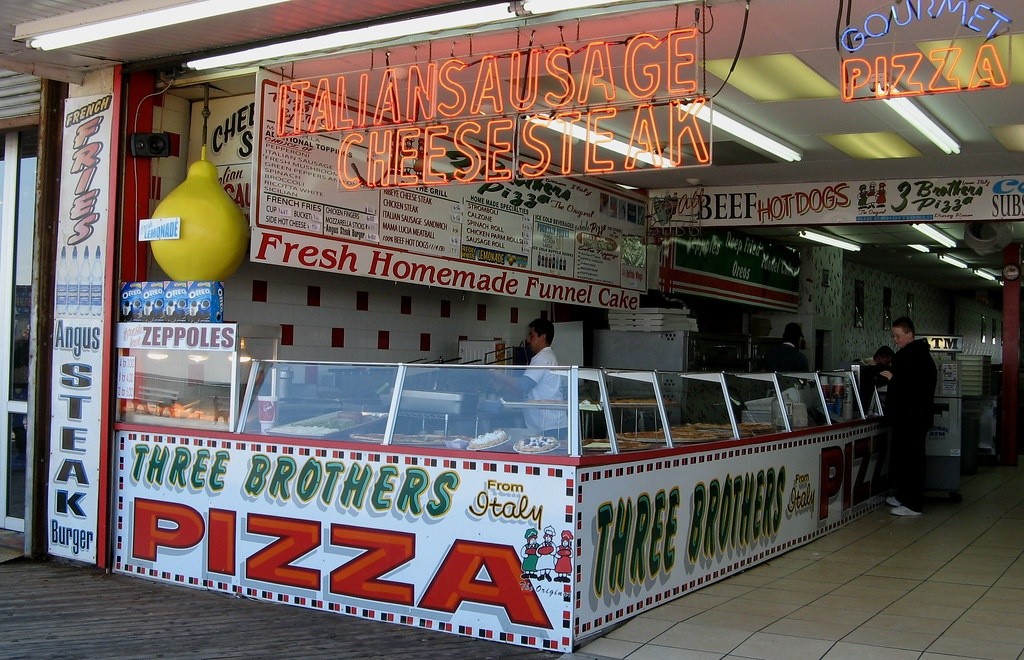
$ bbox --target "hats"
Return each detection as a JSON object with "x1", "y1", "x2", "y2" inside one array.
[{"x1": 784, "y1": 323, "x2": 804, "y2": 338}]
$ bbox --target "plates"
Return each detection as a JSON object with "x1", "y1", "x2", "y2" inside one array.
[
  {"x1": 513, "y1": 441, "x2": 561, "y2": 454},
  {"x1": 466, "y1": 434, "x2": 512, "y2": 450}
]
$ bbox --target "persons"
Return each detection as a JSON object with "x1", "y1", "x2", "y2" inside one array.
[
  {"x1": 880, "y1": 316, "x2": 938, "y2": 515},
  {"x1": 766, "y1": 322, "x2": 810, "y2": 373},
  {"x1": 860, "y1": 346, "x2": 895, "y2": 366},
  {"x1": 489, "y1": 318, "x2": 567, "y2": 439}
]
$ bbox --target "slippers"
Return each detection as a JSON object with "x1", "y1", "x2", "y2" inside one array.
[
  {"x1": 890, "y1": 505, "x2": 922, "y2": 516},
  {"x1": 885, "y1": 496, "x2": 901, "y2": 506}
]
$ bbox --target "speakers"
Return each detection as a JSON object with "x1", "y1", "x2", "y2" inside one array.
[{"x1": 130, "y1": 133, "x2": 169, "y2": 158}]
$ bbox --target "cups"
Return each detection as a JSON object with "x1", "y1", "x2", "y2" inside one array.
[{"x1": 258, "y1": 396, "x2": 279, "y2": 433}]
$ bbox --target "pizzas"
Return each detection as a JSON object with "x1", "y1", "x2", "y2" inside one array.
[
  {"x1": 524, "y1": 399, "x2": 567, "y2": 404},
  {"x1": 582, "y1": 422, "x2": 773, "y2": 449},
  {"x1": 350, "y1": 432, "x2": 469, "y2": 444},
  {"x1": 588, "y1": 399, "x2": 672, "y2": 405}
]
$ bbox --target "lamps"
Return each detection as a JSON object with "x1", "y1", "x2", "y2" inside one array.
[
  {"x1": 972, "y1": 268, "x2": 995, "y2": 281},
  {"x1": 180, "y1": 0, "x2": 619, "y2": 72},
  {"x1": 936, "y1": 253, "x2": 971, "y2": 269},
  {"x1": 187, "y1": 351, "x2": 209, "y2": 363},
  {"x1": 147, "y1": 350, "x2": 170, "y2": 360},
  {"x1": 228, "y1": 338, "x2": 252, "y2": 362},
  {"x1": 795, "y1": 227, "x2": 861, "y2": 252},
  {"x1": 867, "y1": 73, "x2": 962, "y2": 155},
  {"x1": 909, "y1": 223, "x2": 957, "y2": 248},
  {"x1": 673, "y1": 92, "x2": 806, "y2": 164},
  {"x1": 520, "y1": 111, "x2": 678, "y2": 168},
  {"x1": 907, "y1": 245, "x2": 930, "y2": 253},
  {"x1": 12, "y1": 0, "x2": 292, "y2": 52}
]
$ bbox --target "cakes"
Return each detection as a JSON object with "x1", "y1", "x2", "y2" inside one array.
[
  {"x1": 468, "y1": 430, "x2": 507, "y2": 449},
  {"x1": 514, "y1": 436, "x2": 557, "y2": 452}
]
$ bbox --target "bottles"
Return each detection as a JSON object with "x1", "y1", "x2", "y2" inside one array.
[
  {"x1": 653, "y1": 191, "x2": 678, "y2": 226},
  {"x1": 770, "y1": 392, "x2": 793, "y2": 427}
]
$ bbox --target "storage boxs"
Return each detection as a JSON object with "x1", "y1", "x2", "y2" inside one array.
[
  {"x1": 119, "y1": 280, "x2": 225, "y2": 323},
  {"x1": 608, "y1": 308, "x2": 698, "y2": 333},
  {"x1": 955, "y1": 355, "x2": 992, "y2": 395}
]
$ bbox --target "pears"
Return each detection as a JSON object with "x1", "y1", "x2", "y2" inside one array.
[{"x1": 149, "y1": 145, "x2": 248, "y2": 282}]
